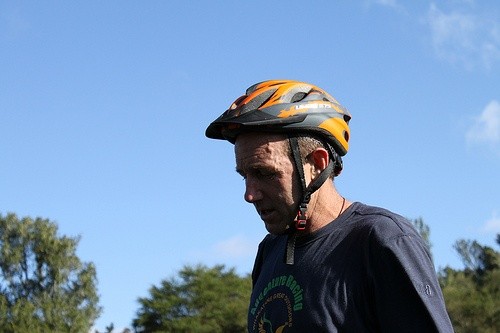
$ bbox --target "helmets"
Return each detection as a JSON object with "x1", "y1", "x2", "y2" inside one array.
[{"x1": 205, "y1": 80, "x2": 352, "y2": 155}]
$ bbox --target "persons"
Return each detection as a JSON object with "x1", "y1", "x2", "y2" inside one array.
[{"x1": 204, "y1": 81, "x2": 455, "y2": 330}]
